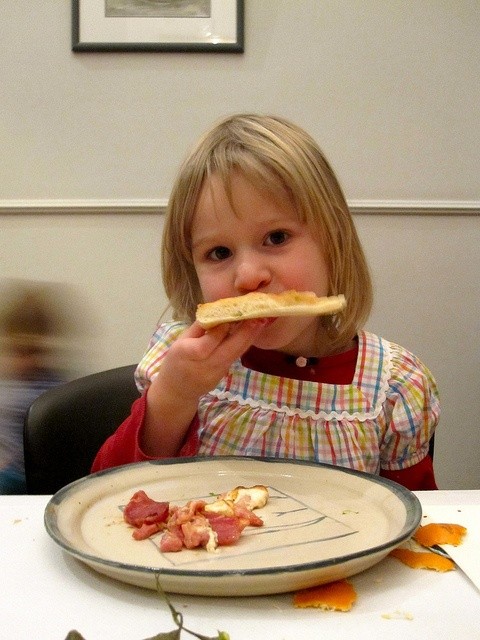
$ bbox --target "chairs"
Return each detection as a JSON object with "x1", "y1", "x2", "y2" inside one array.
[{"x1": 24, "y1": 364, "x2": 434, "y2": 494}]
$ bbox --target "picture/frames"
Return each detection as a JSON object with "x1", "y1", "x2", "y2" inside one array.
[{"x1": 71, "y1": 0, "x2": 245, "y2": 54}]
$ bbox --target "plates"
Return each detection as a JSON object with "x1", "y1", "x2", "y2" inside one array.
[{"x1": 43, "y1": 455, "x2": 423, "y2": 598}]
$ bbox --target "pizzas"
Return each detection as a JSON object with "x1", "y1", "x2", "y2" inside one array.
[{"x1": 196, "y1": 289, "x2": 348, "y2": 328}]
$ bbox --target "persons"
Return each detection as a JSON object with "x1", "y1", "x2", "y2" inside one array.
[{"x1": 89, "y1": 112, "x2": 439, "y2": 490}]
